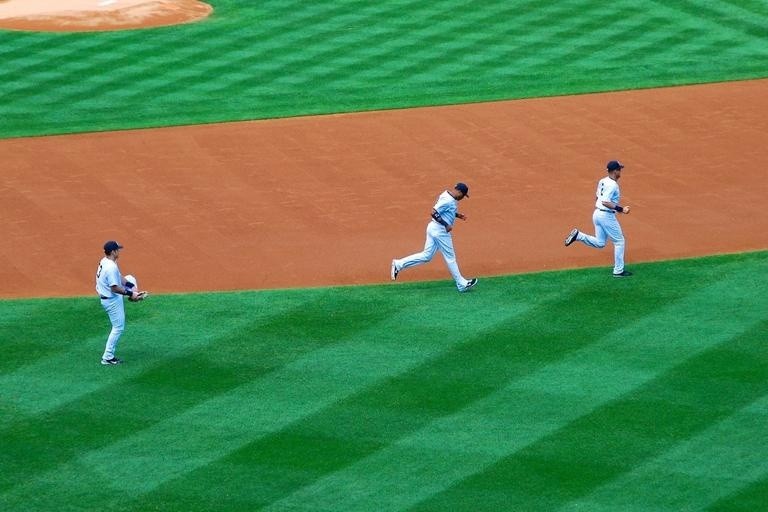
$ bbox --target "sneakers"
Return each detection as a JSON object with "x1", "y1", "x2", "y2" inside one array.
[
  {"x1": 460, "y1": 278, "x2": 479, "y2": 294},
  {"x1": 100, "y1": 358, "x2": 120, "y2": 365},
  {"x1": 129, "y1": 291, "x2": 146, "y2": 302},
  {"x1": 391, "y1": 260, "x2": 400, "y2": 282},
  {"x1": 565, "y1": 228, "x2": 578, "y2": 247},
  {"x1": 614, "y1": 270, "x2": 631, "y2": 277}
]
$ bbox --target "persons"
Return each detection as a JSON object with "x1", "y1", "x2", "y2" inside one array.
[
  {"x1": 96, "y1": 240, "x2": 147, "y2": 365},
  {"x1": 564, "y1": 160, "x2": 634, "y2": 277},
  {"x1": 391, "y1": 182, "x2": 478, "y2": 291}
]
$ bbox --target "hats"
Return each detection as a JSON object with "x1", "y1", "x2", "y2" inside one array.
[
  {"x1": 104, "y1": 241, "x2": 123, "y2": 251},
  {"x1": 454, "y1": 183, "x2": 469, "y2": 198},
  {"x1": 608, "y1": 161, "x2": 624, "y2": 172}
]
